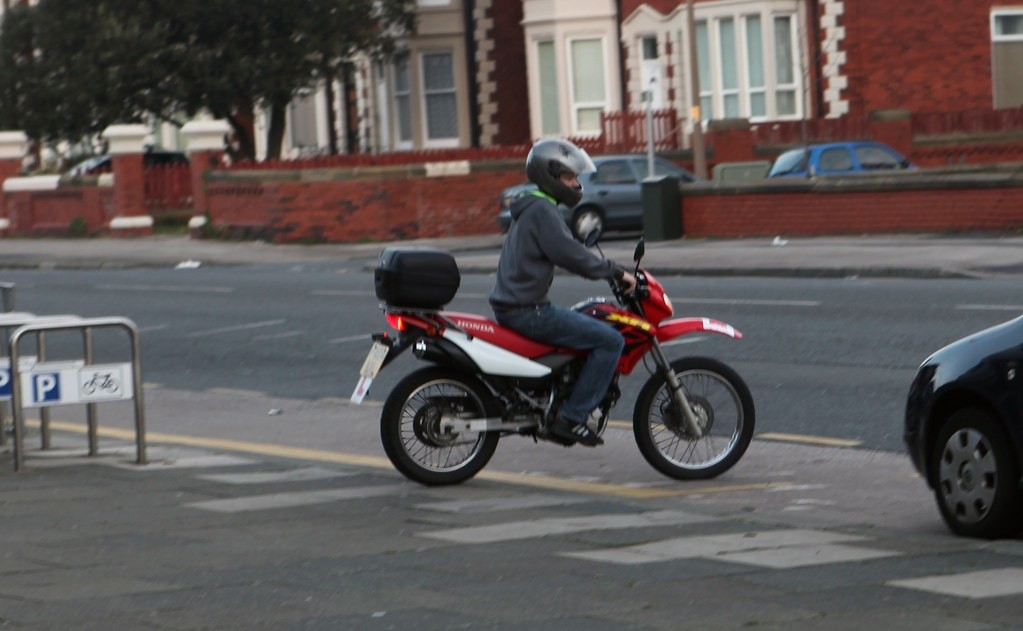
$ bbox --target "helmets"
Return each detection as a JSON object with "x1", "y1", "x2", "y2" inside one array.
[{"x1": 525, "y1": 140, "x2": 585, "y2": 208}]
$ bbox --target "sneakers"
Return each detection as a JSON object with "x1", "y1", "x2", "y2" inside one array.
[{"x1": 548, "y1": 414, "x2": 604, "y2": 446}]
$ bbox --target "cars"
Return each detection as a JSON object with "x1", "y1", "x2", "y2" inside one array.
[
  {"x1": 765, "y1": 139, "x2": 913, "y2": 181},
  {"x1": 901, "y1": 312, "x2": 1022, "y2": 539},
  {"x1": 498, "y1": 152, "x2": 696, "y2": 248},
  {"x1": 62, "y1": 152, "x2": 193, "y2": 227}
]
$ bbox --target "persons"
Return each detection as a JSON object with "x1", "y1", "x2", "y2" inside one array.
[{"x1": 489, "y1": 136, "x2": 637, "y2": 445}]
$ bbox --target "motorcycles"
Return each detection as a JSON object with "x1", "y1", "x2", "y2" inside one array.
[{"x1": 351, "y1": 228, "x2": 754, "y2": 488}]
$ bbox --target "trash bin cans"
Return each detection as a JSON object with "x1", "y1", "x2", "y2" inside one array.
[{"x1": 639, "y1": 173, "x2": 683, "y2": 242}]
